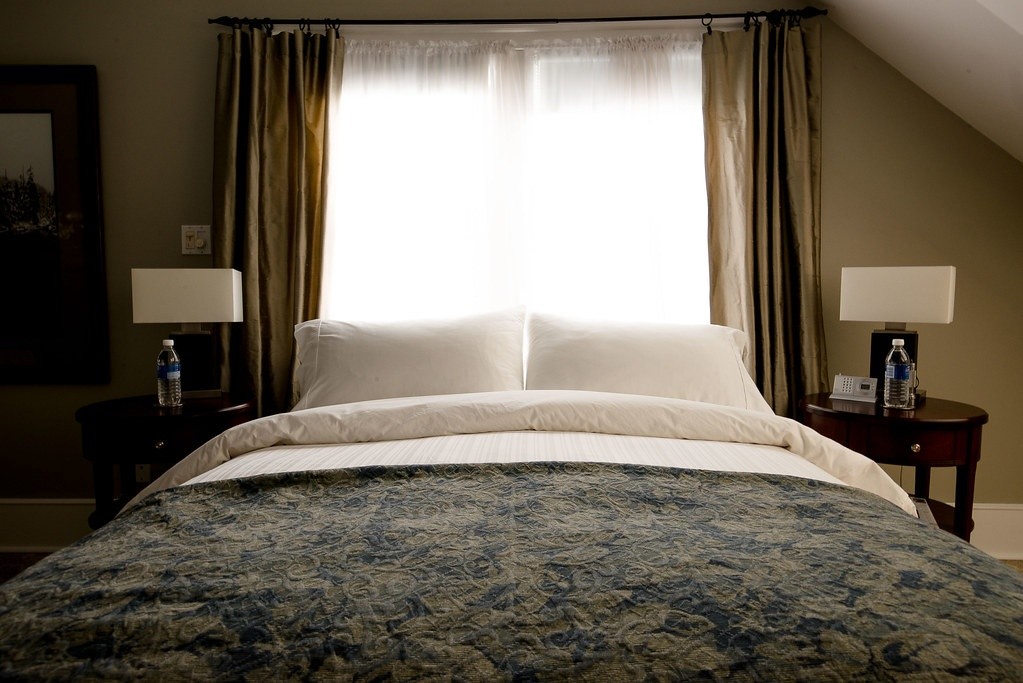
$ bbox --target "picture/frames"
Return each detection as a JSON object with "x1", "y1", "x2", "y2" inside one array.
[{"x1": 0, "y1": 64, "x2": 113, "y2": 389}]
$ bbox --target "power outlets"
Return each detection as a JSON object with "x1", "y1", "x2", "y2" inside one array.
[{"x1": 181, "y1": 225, "x2": 213, "y2": 256}]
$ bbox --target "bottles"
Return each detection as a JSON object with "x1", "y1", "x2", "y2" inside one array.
[
  {"x1": 157, "y1": 339, "x2": 182, "y2": 408},
  {"x1": 883, "y1": 338, "x2": 910, "y2": 407},
  {"x1": 909, "y1": 362, "x2": 915, "y2": 408}
]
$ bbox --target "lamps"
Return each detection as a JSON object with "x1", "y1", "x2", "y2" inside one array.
[
  {"x1": 132, "y1": 268, "x2": 244, "y2": 398},
  {"x1": 839, "y1": 266, "x2": 956, "y2": 402}
]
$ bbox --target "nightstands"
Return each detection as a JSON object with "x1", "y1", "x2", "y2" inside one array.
[
  {"x1": 798, "y1": 393, "x2": 990, "y2": 544},
  {"x1": 76, "y1": 395, "x2": 250, "y2": 534}
]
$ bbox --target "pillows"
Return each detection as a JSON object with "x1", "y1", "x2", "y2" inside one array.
[
  {"x1": 288, "y1": 303, "x2": 526, "y2": 413},
  {"x1": 526, "y1": 310, "x2": 776, "y2": 417}
]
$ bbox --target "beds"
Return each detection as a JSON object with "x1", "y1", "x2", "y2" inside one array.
[{"x1": 0, "y1": 389, "x2": 1023, "y2": 683}]
